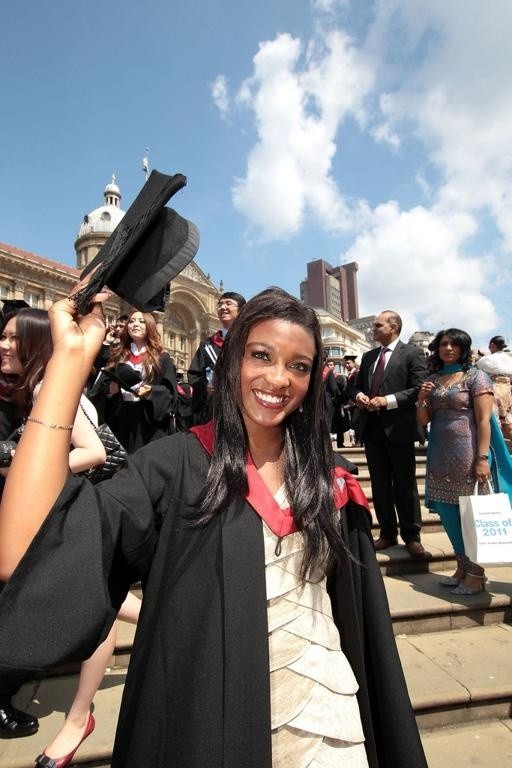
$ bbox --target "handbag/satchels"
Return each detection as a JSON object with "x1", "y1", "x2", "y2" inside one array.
[
  {"x1": 66, "y1": 402, "x2": 133, "y2": 493},
  {"x1": 458, "y1": 472, "x2": 512, "y2": 569}
]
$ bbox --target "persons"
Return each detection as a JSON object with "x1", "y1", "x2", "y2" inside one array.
[
  {"x1": 187, "y1": 292, "x2": 245, "y2": 421},
  {"x1": 109, "y1": 311, "x2": 177, "y2": 452},
  {"x1": 0, "y1": 297, "x2": 141, "y2": 766},
  {"x1": 172, "y1": 373, "x2": 193, "y2": 431},
  {"x1": 323, "y1": 309, "x2": 512, "y2": 596},
  {"x1": 0, "y1": 259, "x2": 428, "y2": 767}
]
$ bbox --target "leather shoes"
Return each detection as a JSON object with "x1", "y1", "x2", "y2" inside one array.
[
  {"x1": 438, "y1": 575, "x2": 460, "y2": 588},
  {"x1": 373, "y1": 535, "x2": 399, "y2": 551},
  {"x1": 404, "y1": 541, "x2": 425, "y2": 556},
  {"x1": 0, "y1": 694, "x2": 40, "y2": 739},
  {"x1": 35, "y1": 708, "x2": 96, "y2": 768},
  {"x1": 449, "y1": 579, "x2": 485, "y2": 595}
]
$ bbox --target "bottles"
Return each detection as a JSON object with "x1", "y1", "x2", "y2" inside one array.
[{"x1": 205, "y1": 366, "x2": 214, "y2": 384}]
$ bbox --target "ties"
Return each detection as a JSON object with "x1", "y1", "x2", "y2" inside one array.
[{"x1": 364, "y1": 349, "x2": 392, "y2": 401}]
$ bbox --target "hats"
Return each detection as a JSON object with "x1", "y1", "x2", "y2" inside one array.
[
  {"x1": 97, "y1": 362, "x2": 146, "y2": 401},
  {"x1": 67, "y1": 166, "x2": 208, "y2": 325},
  {"x1": 341, "y1": 353, "x2": 358, "y2": 362}
]
